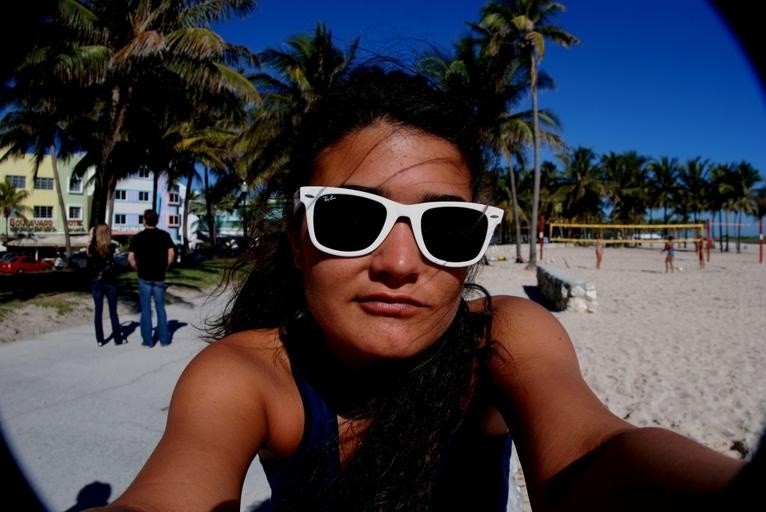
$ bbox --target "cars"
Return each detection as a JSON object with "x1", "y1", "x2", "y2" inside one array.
[{"x1": 0, "y1": 255, "x2": 55, "y2": 274}]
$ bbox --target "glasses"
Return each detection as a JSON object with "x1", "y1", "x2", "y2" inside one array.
[{"x1": 295, "y1": 185, "x2": 505, "y2": 270}]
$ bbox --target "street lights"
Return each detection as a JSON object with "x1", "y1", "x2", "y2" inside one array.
[{"x1": 239, "y1": 180, "x2": 250, "y2": 254}]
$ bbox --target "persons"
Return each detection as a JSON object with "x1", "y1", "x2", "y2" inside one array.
[
  {"x1": 84, "y1": 222, "x2": 130, "y2": 345},
  {"x1": 595, "y1": 231, "x2": 603, "y2": 269},
  {"x1": 662, "y1": 235, "x2": 675, "y2": 274},
  {"x1": 63, "y1": 51, "x2": 754, "y2": 512},
  {"x1": 124, "y1": 208, "x2": 178, "y2": 347}
]
